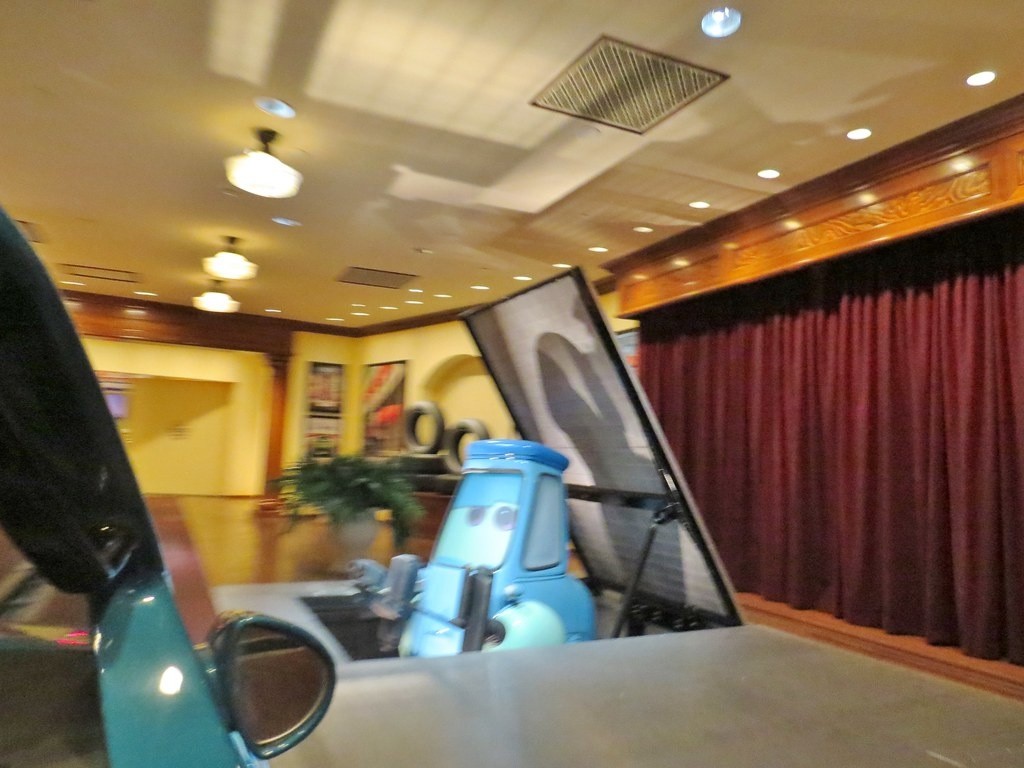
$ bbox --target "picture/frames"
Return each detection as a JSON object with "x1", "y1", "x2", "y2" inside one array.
[
  {"x1": 310, "y1": 362, "x2": 345, "y2": 412},
  {"x1": 302, "y1": 416, "x2": 341, "y2": 458},
  {"x1": 362, "y1": 359, "x2": 406, "y2": 452}
]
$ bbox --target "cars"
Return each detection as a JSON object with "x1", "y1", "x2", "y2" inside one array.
[{"x1": 0, "y1": 211, "x2": 339, "y2": 767}]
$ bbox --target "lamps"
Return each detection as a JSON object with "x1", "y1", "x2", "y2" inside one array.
[
  {"x1": 191, "y1": 282, "x2": 239, "y2": 313},
  {"x1": 202, "y1": 237, "x2": 259, "y2": 281},
  {"x1": 224, "y1": 127, "x2": 302, "y2": 199}
]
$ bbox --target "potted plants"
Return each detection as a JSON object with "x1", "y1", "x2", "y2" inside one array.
[{"x1": 262, "y1": 450, "x2": 428, "y2": 548}]
